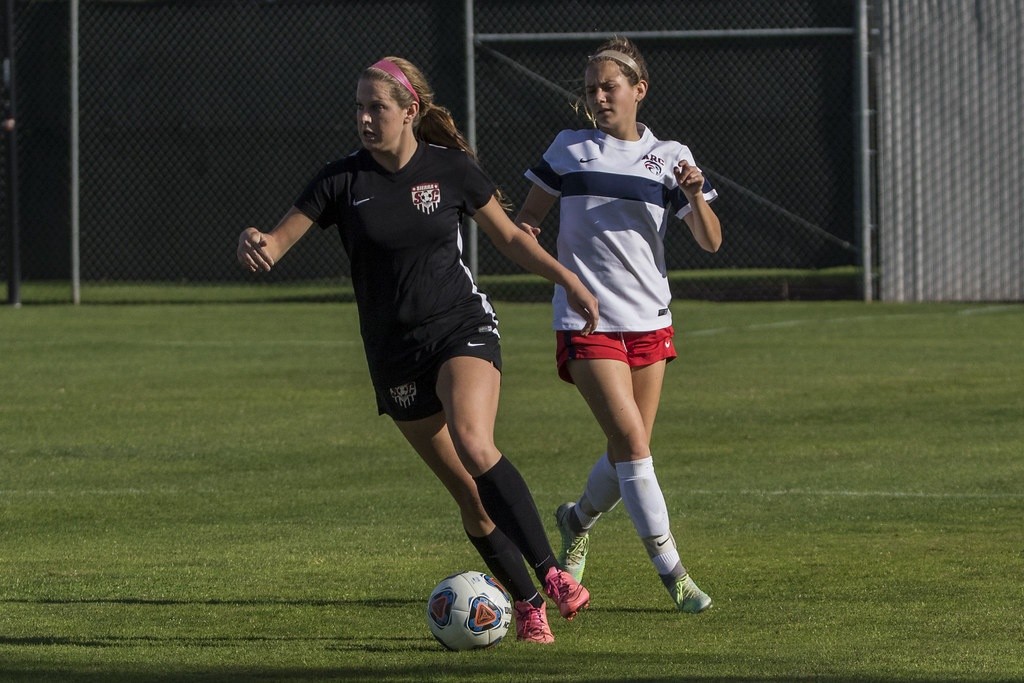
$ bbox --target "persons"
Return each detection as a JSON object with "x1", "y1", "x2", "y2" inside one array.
[
  {"x1": 233, "y1": 52, "x2": 601, "y2": 649},
  {"x1": 505, "y1": 29, "x2": 728, "y2": 616}
]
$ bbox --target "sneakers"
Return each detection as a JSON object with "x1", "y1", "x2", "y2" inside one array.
[
  {"x1": 543, "y1": 567, "x2": 590, "y2": 621},
  {"x1": 666, "y1": 571, "x2": 712, "y2": 614},
  {"x1": 554, "y1": 501, "x2": 589, "y2": 584},
  {"x1": 512, "y1": 600, "x2": 555, "y2": 643}
]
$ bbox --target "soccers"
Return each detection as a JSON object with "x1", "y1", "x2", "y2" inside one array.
[{"x1": 427, "y1": 571, "x2": 511, "y2": 652}]
